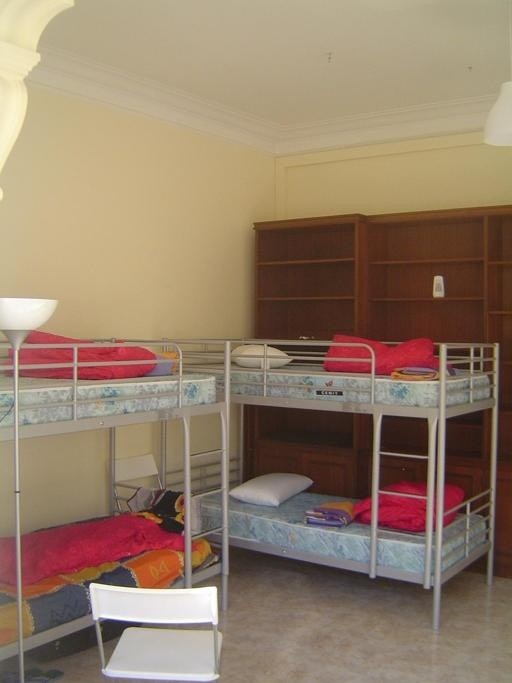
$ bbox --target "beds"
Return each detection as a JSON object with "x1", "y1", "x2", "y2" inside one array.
[
  {"x1": 164, "y1": 335, "x2": 500, "y2": 631},
  {"x1": 0, "y1": 338, "x2": 233, "y2": 682}
]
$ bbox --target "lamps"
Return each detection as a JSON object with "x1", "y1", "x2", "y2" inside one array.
[
  {"x1": 0, "y1": 296, "x2": 61, "y2": 683},
  {"x1": 482, "y1": 79, "x2": 512, "y2": 148}
]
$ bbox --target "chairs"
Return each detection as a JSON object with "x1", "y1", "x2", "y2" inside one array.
[
  {"x1": 102, "y1": 451, "x2": 166, "y2": 514},
  {"x1": 87, "y1": 582, "x2": 223, "y2": 682}
]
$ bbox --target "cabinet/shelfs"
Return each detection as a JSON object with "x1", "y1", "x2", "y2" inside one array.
[{"x1": 244, "y1": 202, "x2": 511, "y2": 578}]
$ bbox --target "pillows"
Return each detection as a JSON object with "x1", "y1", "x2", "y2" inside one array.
[
  {"x1": 229, "y1": 470, "x2": 314, "y2": 508},
  {"x1": 232, "y1": 342, "x2": 289, "y2": 366}
]
